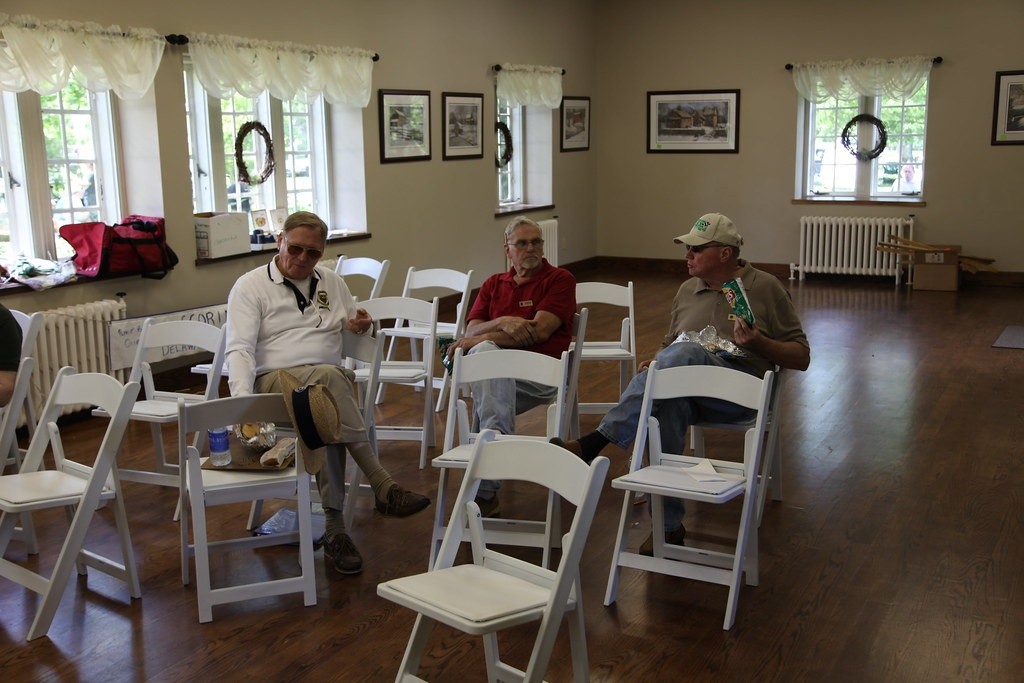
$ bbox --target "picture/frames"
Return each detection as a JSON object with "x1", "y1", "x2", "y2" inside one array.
[
  {"x1": 559, "y1": 95, "x2": 590, "y2": 153},
  {"x1": 441, "y1": 91, "x2": 484, "y2": 161},
  {"x1": 991, "y1": 71, "x2": 1024, "y2": 147},
  {"x1": 645, "y1": 89, "x2": 741, "y2": 154},
  {"x1": 377, "y1": 88, "x2": 432, "y2": 165}
]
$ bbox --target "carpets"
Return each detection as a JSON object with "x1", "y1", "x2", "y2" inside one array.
[{"x1": 991, "y1": 325, "x2": 1024, "y2": 348}]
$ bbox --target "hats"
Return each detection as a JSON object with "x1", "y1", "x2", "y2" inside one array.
[
  {"x1": 673, "y1": 213, "x2": 744, "y2": 247},
  {"x1": 277, "y1": 368, "x2": 341, "y2": 474}
]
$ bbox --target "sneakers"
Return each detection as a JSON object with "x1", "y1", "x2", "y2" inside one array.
[
  {"x1": 323, "y1": 532, "x2": 363, "y2": 575},
  {"x1": 374, "y1": 483, "x2": 433, "y2": 520}
]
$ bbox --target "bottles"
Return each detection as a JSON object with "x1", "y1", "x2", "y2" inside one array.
[{"x1": 208, "y1": 425, "x2": 231, "y2": 466}]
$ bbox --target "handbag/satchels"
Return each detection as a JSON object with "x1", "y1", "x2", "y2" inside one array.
[{"x1": 59, "y1": 214, "x2": 180, "y2": 280}]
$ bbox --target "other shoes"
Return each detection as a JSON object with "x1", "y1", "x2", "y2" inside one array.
[
  {"x1": 639, "y1": 523, "x2": 686, "y2": 556},
  {"x1": 474, "y1": 491, "x2": 501, "y2": 518},
  {"x1": 549, "y1": 437, "x2": 593, "y2": 466}
]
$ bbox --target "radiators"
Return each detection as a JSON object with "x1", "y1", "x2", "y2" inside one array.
[
  {"x1": 790, "y1": 215, "x2": 914, "y2": 285},
  {"x1": 506, "y1": 219, "x2": 558, "y2": 274},
  {"x1": 17, "y1": 292, "x2": 130, "y2": 429}
]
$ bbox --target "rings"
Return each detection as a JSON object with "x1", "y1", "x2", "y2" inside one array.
[{"x1": 357, "y1": 329, "x2": 363, "y2": 334}]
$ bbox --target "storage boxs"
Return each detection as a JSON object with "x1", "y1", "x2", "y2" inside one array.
[
  {"x1": 193, "y1": 211, "x2": 252, "y2": 259},
  {"x1": 914, "y1": 244, "x2": 961, "y2": 291}
]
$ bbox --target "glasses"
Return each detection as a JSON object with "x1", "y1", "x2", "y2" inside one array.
[
  {"x1": 284, "y1": 233, "x2": 324, "y2": 260},
  {"x1": 507, "y1": 238, "x2": 544, "y2": 250},
  {"x1": 302, "y1": 294, "x2": 323, "y2": 328},
  {"x1": 686, "y1": 243, "x2": 732, "y2": 253}
]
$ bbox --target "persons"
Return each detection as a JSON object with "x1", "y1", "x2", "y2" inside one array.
[
  {"x1": 814, "y1": 149, "x2": 825, "y2": 185},
  {"x1": 0, "y1": 301, "x2": 23, "y2": 408},
  {"x1": 71, "y1": 163, "x2": 99, "y2": 222},
  {"x1": 890, "y1": 165, "x2": 919, "y2": 192},
  {"x1": 317, "y1": 291, "x2": 329, "y2": 307},
  {"x1": 223, "y1": 211, "x2": 432, "y2": 575},
  {"x1": 446, "y1": 215, "x2": 576, "y2": 517},
  {"x1": 548, "y1": 212, "x2": 811, "y2": 555}
]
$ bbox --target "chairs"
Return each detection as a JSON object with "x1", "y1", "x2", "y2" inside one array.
[{"x1": 0, "y1": 257, "x2": 785, "y2": 683}]
